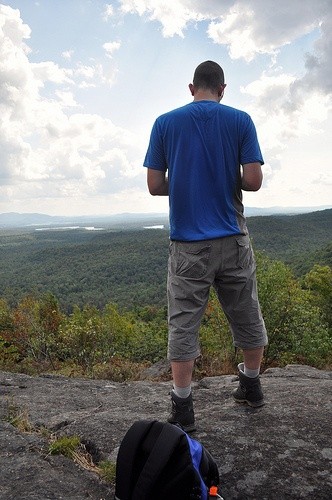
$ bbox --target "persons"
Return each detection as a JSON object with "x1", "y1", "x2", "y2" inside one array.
[{"x1": 143, "y1": 61, "x2": 269, "y2": 432}]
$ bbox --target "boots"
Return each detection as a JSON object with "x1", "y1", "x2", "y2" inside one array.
[
  {"x1": 164, "y1": 388, "x2": 196, "y2": 432},
  {"x1": 231, "y1": 362, "x2": 265, "y2": 408}
]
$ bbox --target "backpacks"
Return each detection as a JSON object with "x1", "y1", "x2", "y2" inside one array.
[{"x1": 114, "y1": 418, "x2": 223, "y2": 500}]
